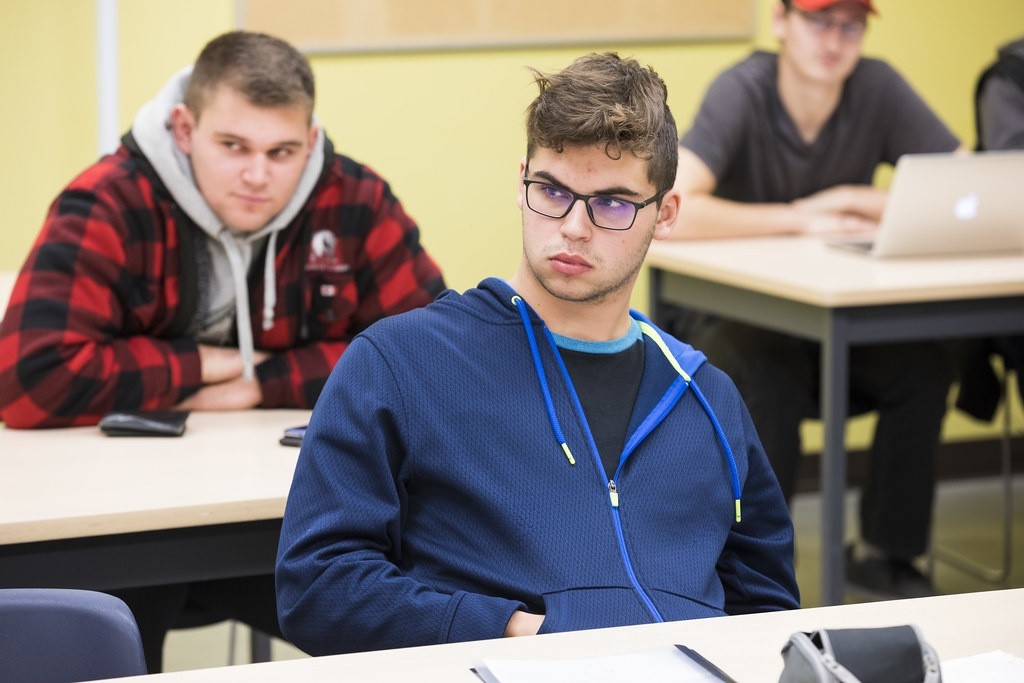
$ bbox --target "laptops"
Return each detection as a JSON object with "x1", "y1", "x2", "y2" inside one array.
[{"x1": 826, "y1": 149, "x2": 1024, "y2": 257}]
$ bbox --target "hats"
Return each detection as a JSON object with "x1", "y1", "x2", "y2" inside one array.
[{"x1": 789, "y1": 0, "x2": 882, "y2": 18}]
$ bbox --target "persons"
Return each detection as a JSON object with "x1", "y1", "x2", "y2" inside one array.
[
  {"x1": 660, "y1": 0, "x2": 972, "y2": 600},
  {"x1": 0, "y1": 32, "x2": 446, "y2": 673},
  {"x1": 275, "y1": 53, "x2": 802, "y2": 654}
]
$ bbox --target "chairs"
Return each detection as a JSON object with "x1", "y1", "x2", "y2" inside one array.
[{"x1": 0, "y1": 587, "x2": 148, "y2": 683}]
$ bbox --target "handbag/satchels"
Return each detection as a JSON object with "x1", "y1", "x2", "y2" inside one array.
[{"x1": 778, "y1": 626, "x2": 943, "y2": 683}]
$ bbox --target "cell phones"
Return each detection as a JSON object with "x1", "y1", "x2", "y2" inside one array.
[{"x1": 284, "y1": 425, "x2": 308, "y2": 438}]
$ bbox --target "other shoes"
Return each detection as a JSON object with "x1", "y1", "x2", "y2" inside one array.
[{"x1": 844, "y1": 540, "x2": 935, "y2": 598}]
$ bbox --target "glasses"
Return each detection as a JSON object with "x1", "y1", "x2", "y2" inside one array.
[
  {"x1": 789, "y1": 6, "x2": 867, "y2": 37},
  {"x1": 523, "y1": 162, "x2": 671, "y2": 230}
]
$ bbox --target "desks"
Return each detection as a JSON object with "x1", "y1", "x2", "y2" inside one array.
[
  {"x1": 0, "y1": 409, "x2": 313, "y2": 663},
  {"x1": 649, "y1": 235, "x2": 1024, "y2": 606},
  {"x1": 108, "y1": 586, "x2": 1023, "y2": 683}
]
